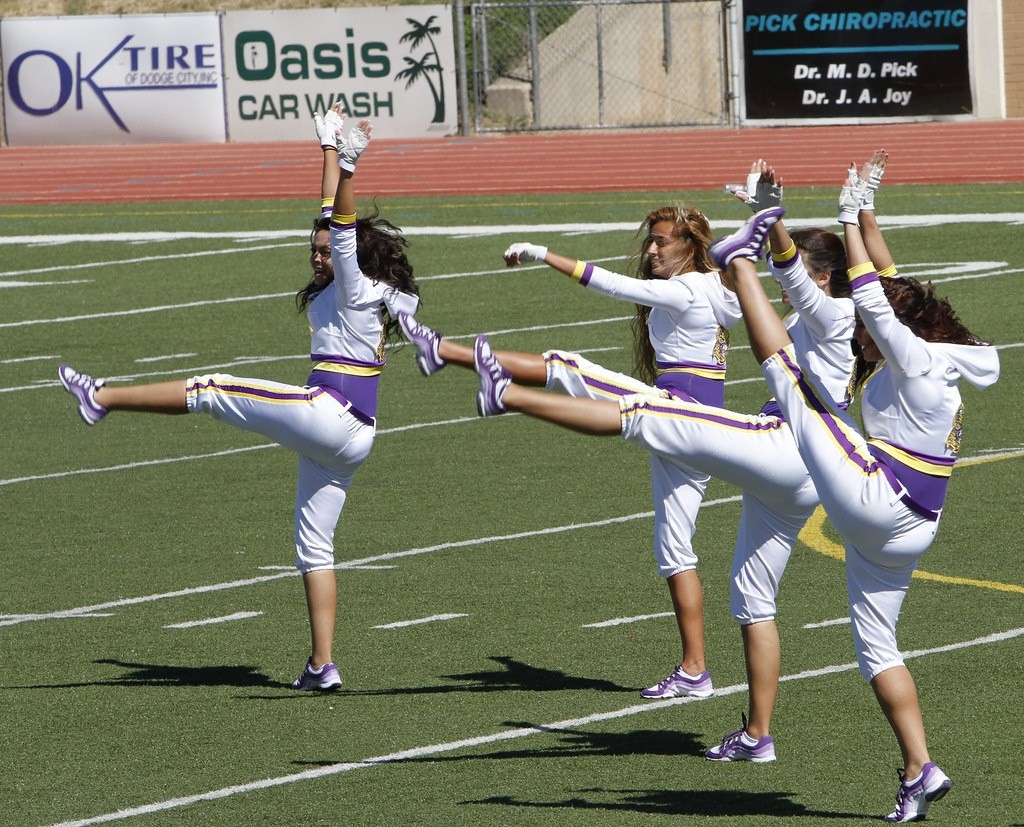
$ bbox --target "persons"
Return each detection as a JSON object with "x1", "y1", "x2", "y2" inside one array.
[
  {"x1": 396, "y1": 147, "x2": 1001, "y2": 824},
  {"x1": 57, "y1": 101, "x2": 425, "y2": 692}
]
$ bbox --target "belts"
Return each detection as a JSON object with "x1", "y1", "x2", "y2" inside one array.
[
  {"x1": 879, "y1": 464, "x2": 938, "y2": 521},
  {"x1": 667, "y1": 385, "x2": 696, "y2": 405},
  {"x1": 322, "y1": 387, "x2": 375, "y2": 427}
]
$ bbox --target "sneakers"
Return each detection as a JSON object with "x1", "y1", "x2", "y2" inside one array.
[
  {"x1": 473, "y1": 334, "x2": 513, "y2": 417},
  {"x1": 706, "y1": 205, "x2": 786, "y2": 272},
  {"x1": 884, "y1": 762, "x2": 951, "y2": 824},
  {"x1": 396, "y1": 310, "x2": 447, "y2": 377},
  {"x1": 704, "y1": 712, "x2": 776, "y2": 764},
  {"x1": 58, "y1": 364, "x2": 110, "y2": 426},
  {"x1": 640, "y1": 664, "x2": 715, "y2": 699},
  {"x1": 291, "y1": 656, "x2": 342, "y2": 691}
]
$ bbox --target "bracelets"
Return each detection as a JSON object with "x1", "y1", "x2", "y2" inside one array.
[{"x1": 323, "y1": 147, "x2": 337, "y2": 152}]
